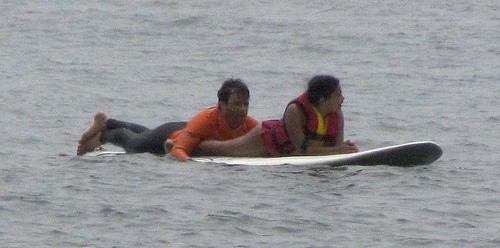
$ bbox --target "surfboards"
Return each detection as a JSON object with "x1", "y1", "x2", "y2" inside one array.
[{"x1": 81, "y1": 141, "x2": 442, "y2": 169}]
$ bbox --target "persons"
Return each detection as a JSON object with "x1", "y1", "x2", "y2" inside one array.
[
  {"x1": 76, "y1": 75, "x2": 262, "y2": 165},
  {"x1": 164, "y1": 74, "x2": 361, "y2": 162}
]
